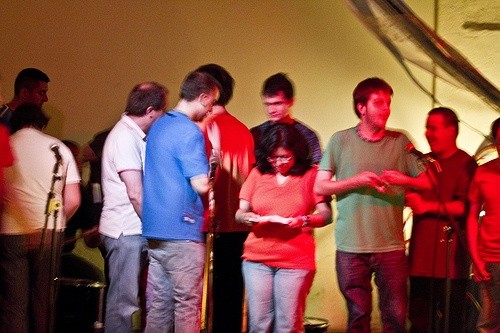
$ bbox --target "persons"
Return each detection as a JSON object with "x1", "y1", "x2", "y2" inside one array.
[
  {"x1": 405, "y1": 108, "x2": 478, "y2": 333},
  {"x1": 313, "y1": 77, "x2": 433, "y2": 333},
  {"x1": 0, "y1": 68, "x2": 116, "y2": 333},
  {"x1": 142, "y1": 70, "x2": 220, "y2": 333},
  {"x1": 196, "y1": 63, "x2": 323, "y2": 333},
  {"x1": 99, "y1": 81, "x2": 169, "y2": 333},
  {"x1": 236, "y1": 123, "x2": 333, "y2": 333},
  {"x1": 466, "y1": 118, "x2": 500, "y2": 333}
]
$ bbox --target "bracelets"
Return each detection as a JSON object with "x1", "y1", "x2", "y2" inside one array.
[{"x1": 301, "y1": 215, "x2": 310, "y2": 228}]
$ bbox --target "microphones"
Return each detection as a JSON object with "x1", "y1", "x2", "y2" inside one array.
[
  {"x1": 405, "y1": 144, "x2": 435, "y2": 162},
  {"x1": 51, "y1": 144, "x2": 62, "y2": 163},
  {"x1": 210, "y1": 156, "x2": 220, "y2": 180}
]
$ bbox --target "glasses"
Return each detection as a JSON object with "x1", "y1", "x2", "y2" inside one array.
[{"x1": 267, "y1": 155, "x2": 294, "y2": 163}]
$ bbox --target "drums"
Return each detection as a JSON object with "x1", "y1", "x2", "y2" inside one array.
[{"x1": 272, "y1": 317, "x2": 329, "y2": 333}]
